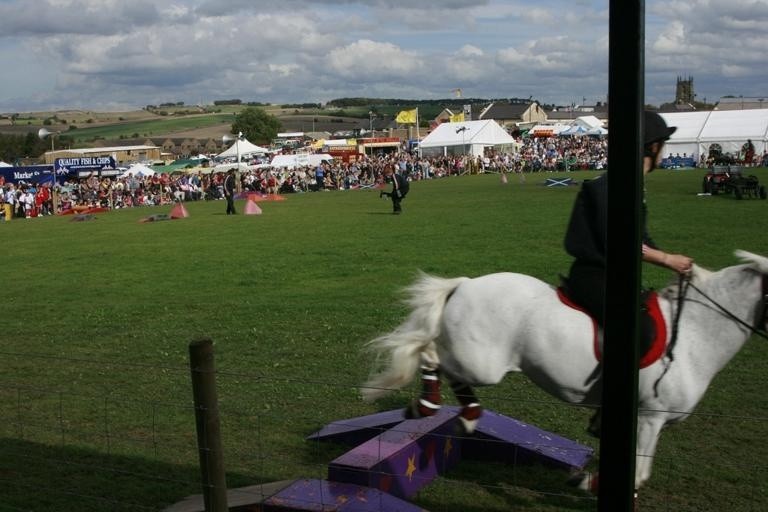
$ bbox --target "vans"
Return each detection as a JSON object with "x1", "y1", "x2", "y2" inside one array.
[{"x1": 271, "y1": 153, "x2": 336, "y2": 168}]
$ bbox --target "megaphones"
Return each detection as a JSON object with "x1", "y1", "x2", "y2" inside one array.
[
  {"x1": 359, "y1": 128, "x2": 372, "y2": 136},
  {"x1": 455, "y1": 128, "x2": 463, "y2": 134},
  {"x1": 38, "y1": 127, "x2": 61, "y2": 141},
  {"x1": 222, "y1": 135, "x2": 237, "y2": 144}
]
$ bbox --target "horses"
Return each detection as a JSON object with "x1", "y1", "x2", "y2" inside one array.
[{"x1": 358, "y1": 249, "x2": 768, "y2": 498}]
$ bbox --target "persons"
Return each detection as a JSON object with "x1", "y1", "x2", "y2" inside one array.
[
  {"x1": 692, "y1": 154, "x2": 697, "y2": 168},
  {"x1": 675, "y1": 153, "x2": 681, "y2": 158},
  {"x1": 668, "y1": 153, "x2": 674, "y2": 159},
  {"x1": 702, "y1": 139, "x2": 768, "y2": 167},
  {"x1": 561, "y1": 113, "x2": 692, "y2": 439},
  {"x1": 682, "y1": 153, "x2": 688, "y2": 158}
]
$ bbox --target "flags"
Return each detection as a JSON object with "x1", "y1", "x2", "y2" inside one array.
[
  {"x1": 450, "y1": 112, "x2": 464, "y2": 123},
  {"x1": 395, "y1": 108, "x2": 417, "y2": 124}
]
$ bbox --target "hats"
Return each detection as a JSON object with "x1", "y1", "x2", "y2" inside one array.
[
  {"x1": 228, "y1": 167, "x2": 238, "y2": 174},
  {"x1": 640, "y1": 109, "x2": 677, "y2": 144}
]
